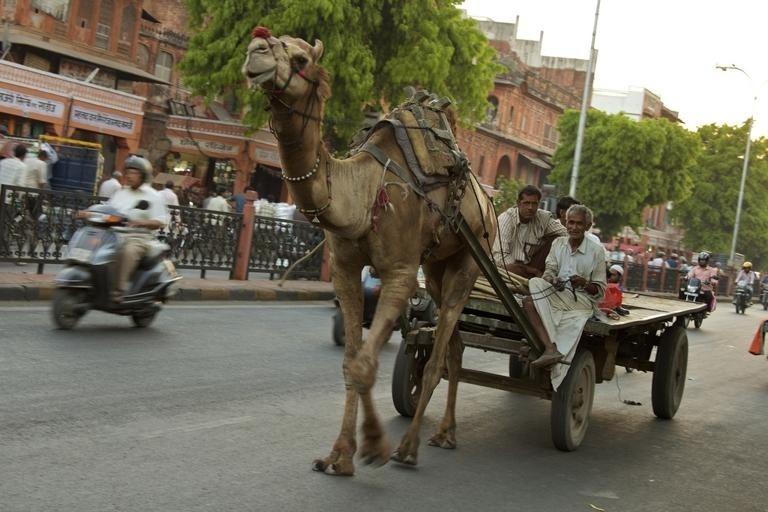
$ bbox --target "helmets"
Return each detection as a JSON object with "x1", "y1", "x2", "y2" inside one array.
[
  {"x1": 123, "y1": 155, "x2": 153, "y2": 182},
  {"x1": 742, "y1": 261, "x2": 752, "y2": 270},
  {"x1": 697, "y1": 251, "x2": 709, "y2": 267}
]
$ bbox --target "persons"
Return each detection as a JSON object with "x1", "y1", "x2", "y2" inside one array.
[
  {"x1": 606, "y1": 246, "x2": 768, "y2": 313},
  {"x1": 79, "y1": 157, "x2": 180, "y2": 298},
  {"x1": 1, "y1": 145, "x2": 49, "y2": 256},
  {"x1": 205, "y1": 190, "x2": 308, "y2": 267},
  {"x1": 491, "y1": 186, "x2": 606, "y2": 390}
]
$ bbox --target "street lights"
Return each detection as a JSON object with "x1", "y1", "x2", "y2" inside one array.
[{"x1": 712, "y1": 60, "x2": 761, "y2": 272}]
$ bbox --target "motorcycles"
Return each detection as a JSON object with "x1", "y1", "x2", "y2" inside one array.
[
  {"x1": 49, "y1": 187, "x2": 182, "y2": 335},
  {"x1": 677, "y1": 273, "x2": 767, "y2": 333},
  {"x1": 330, "y1": 265, "x2": 400, "y2": 348}
]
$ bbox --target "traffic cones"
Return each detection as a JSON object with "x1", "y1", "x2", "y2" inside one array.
[{"x1": 746, "y1": 325, "x2": 761, "y2": 355}]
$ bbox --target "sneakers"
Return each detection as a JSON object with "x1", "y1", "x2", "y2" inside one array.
[{"x1": 530, "y1": 343, "x2": 564, "y2": 367}]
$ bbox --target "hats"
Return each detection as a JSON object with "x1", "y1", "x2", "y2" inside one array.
[{"x1": 610, "y1": 264, "x2": 624, "y2": 276}]
